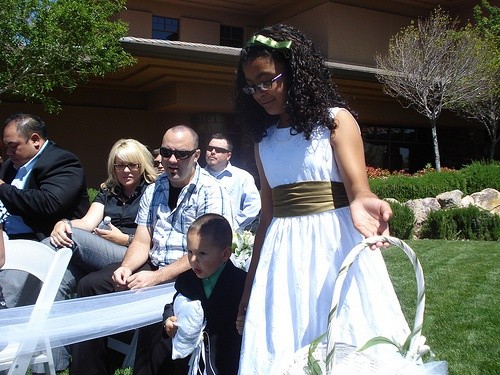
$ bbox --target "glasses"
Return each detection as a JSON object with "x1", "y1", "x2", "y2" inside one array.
[
  {"x1": 206, "y1": 146, "x2": 232, "y2": 153},
  {"x1": 114, "y1": 163, "x2": 139, "y2": 169},
  {"x1": 242, "y1": 73, "x2": 283, "y2": 96},
  {"x1": 160, "y1": 148, "x2": 196, "y2": 160},
  {"x1": 153, "y1": 160, "x2": 163, "y2": 167}
]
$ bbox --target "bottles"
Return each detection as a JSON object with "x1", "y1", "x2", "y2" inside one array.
[{"x1": 94, "y1": 216, "x2": 112, "y2": 234}]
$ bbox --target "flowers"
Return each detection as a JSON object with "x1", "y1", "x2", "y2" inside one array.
[
  {"x1": 232, "y1": 230, "x2": 256, "y2": 270},
  {"x1": 356, "y1": 325, "x2": 435, "y2": 366}
]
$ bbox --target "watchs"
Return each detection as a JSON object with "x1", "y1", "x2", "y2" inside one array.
[{"x1": 61, "y1": 218, "x2": 72, "y2": 229}]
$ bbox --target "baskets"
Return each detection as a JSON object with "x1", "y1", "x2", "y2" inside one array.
[{"x1": 283, "y1": 236, "x2": 448, "y2": 375}]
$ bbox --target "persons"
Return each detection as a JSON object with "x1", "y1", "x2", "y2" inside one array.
[
  {"x1": 0, "y1": 113, "x2": 91, "y2": 242},
  {"x1": 204, "y1": 132, "x2": 262, "y2": 232},
  {"x1": 162, "y1": 213, "x2": 248, "y2": 375},
  {"x1": 77, "y1": 125, "x2": 233, "y2": 298},
  {"x1": 151, "y1": 148, "x2": 164, "y2": 176},
  {"x1": 40, "y1": 138, "x2": 158, "y2": 301},
  {"x1": 236, "y1": 24, "x2": 394, "y2": 375}
]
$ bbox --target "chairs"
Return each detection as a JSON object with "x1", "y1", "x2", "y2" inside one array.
[{"x1": 0, "y1": 231, "x2": 73, "y2": 375}]
